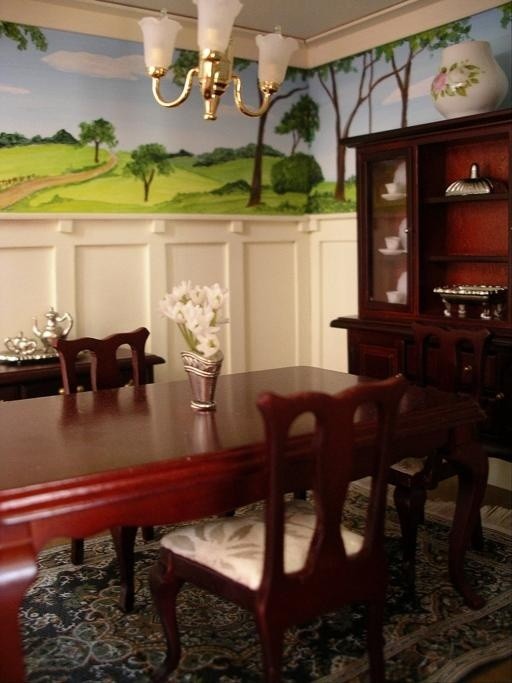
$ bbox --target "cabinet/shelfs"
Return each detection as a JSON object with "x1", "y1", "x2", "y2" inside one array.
[{"x1": 327, "y1": 106, "x2": 512, "y2": 465}]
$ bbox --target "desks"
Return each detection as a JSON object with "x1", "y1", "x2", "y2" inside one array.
[{"x1": 1, "y1": 342, "x2": 166, "y2": 402}]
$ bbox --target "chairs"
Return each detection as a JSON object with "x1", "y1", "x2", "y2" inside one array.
[
  {"x1": 365, "y1": 323, "x2": 493, "y2": 612},
  {"x1": 147, "y1": 374, "x2": 402, "y2": 680},
  {"x1": 50, "y1": 328, "x2": 152, "y2": 614}
]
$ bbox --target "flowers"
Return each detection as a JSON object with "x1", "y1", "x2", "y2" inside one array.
[{"x1": 158, "y1": 281, "x2": 237, "y2": 363}]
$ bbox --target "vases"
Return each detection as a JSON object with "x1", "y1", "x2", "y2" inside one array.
[
  {"x1": 175, "y1": 350, "x2": 225, "y2": 414},
  {"x1": 431, "y1": 36, "x2": 508, "y2": 119}
]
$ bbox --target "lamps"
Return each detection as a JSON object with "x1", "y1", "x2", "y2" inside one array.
[{"x1": 136, "y1": 0, "x2": 301, "y2": 123}]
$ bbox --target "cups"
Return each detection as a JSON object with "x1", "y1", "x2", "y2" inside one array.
[
  {"x1": 383, "y1": 235, "x2": 401, "y2": 248},
  {"x1": 385, "y1": 290, "x2": 406, "y2": 304},
  {"x1": 384, "y1": 182, "x2": 402, "y2": 192}
]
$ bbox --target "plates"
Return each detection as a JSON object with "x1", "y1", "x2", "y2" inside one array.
[
  {"x1": 397, "y1": 270, "x2": 407, "y2": 294},
  {"x1": 398, "y1": 217, "x2": 407, "y2": 249},
  {"x1": 376, "y1": 247, "x2": 404, "y2": 256},
  {"x1": 392, "y1": 161, "x2": 406, "y2": 190},
  {"x1": 381, "y1": 192, "x2": 406, "y2": 200}
]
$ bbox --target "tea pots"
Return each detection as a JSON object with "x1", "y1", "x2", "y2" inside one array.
[
  {"x1": 31, "y1": 304, "x2": 73, "y2": 354},
  {"x1": 4, "y1": 330, "x2": 38, "y2": 356}
]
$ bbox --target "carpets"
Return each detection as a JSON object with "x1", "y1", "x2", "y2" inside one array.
[{"x1": 18, "y1": 480, "x2": 510, "y2": 682}]
